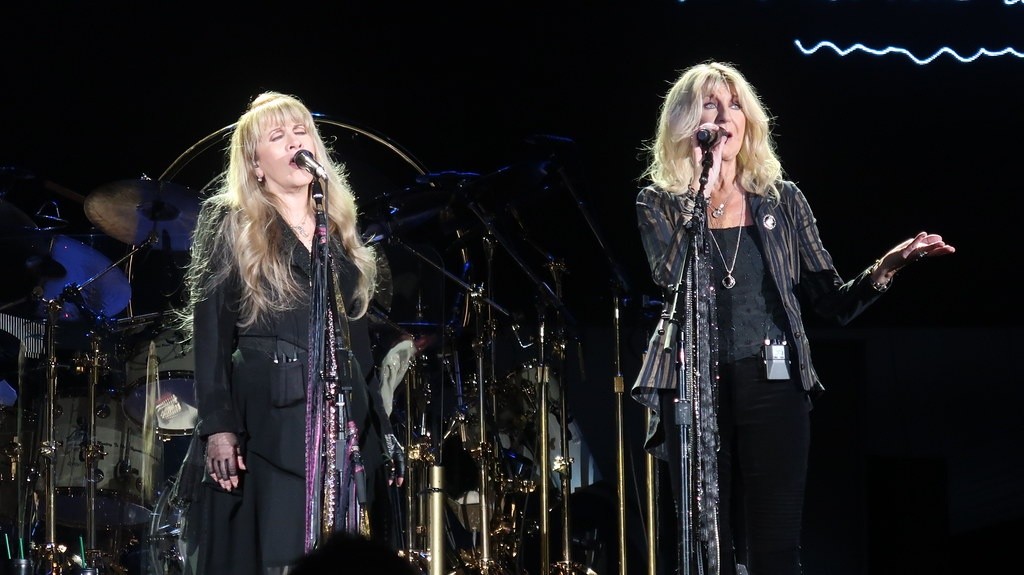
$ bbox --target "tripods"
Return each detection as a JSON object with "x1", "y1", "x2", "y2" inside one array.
[
  {"x1": 0, "y1": 223, "x2": 161, "y2": 575},
  {"x1": 377, "y1": 188, "x2": 598, "y2": 575}
]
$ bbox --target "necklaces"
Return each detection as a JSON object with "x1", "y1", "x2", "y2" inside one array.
[
  {"x1": 287, "y1": 212, "x2": 312, "y2": 239},
  {"x1": 706, "y1": 183, "x2": 746, "y2": 290}
]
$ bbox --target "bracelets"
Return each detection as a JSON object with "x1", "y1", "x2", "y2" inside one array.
[
  {"x1": 870, "y1": 268, "x2": 889, "y2": 292},
  {"x1": 680, "y1": 183, "x2": 697, "y2": 214}
]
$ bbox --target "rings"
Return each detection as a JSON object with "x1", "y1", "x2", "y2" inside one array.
[
  {"x1": 690, "y1": 136, "x2": 693, "y2": 139},
  {"x1": 916, "y1": 250, "x2": 929, "y2": 262}
]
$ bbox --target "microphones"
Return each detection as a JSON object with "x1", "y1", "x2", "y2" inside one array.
[
  {"x1": 698, "y1": 128, "x2": 722, "y2": 148},
  {"x1": 295, "y1": 150, "x2": 329, "y2": 185}
]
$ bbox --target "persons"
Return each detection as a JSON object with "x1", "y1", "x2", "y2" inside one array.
[
  {"x1": 633, "y1": 60, "x2": 959, "y2": 575},
  {"x1": 190, "y1": 87, "x2": 408, "y2": 575}
]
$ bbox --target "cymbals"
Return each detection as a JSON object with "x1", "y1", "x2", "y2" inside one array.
[
  {"x1": 82, "y1": 178, "x2": 204, "y2": 253},
  {"x1": 0, "y1": 199, "x2": 39, "y2": 239},
  {"x1": 31, "y1": 234, "x2": 133, "y2": 320}
]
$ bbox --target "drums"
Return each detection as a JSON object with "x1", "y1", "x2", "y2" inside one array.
[
  {"x1": 497, "y1": 355, "x2": 604, "y2": 514},
  {"x1": 118, "y1": 311, "x2": 200, "y2": 440},
  {"x1": 27, "y1": 387, "x2": 167, "y2": 532},
  {"x1": 132, "y1": 464, "x2": 184, "y2": 574}
]
